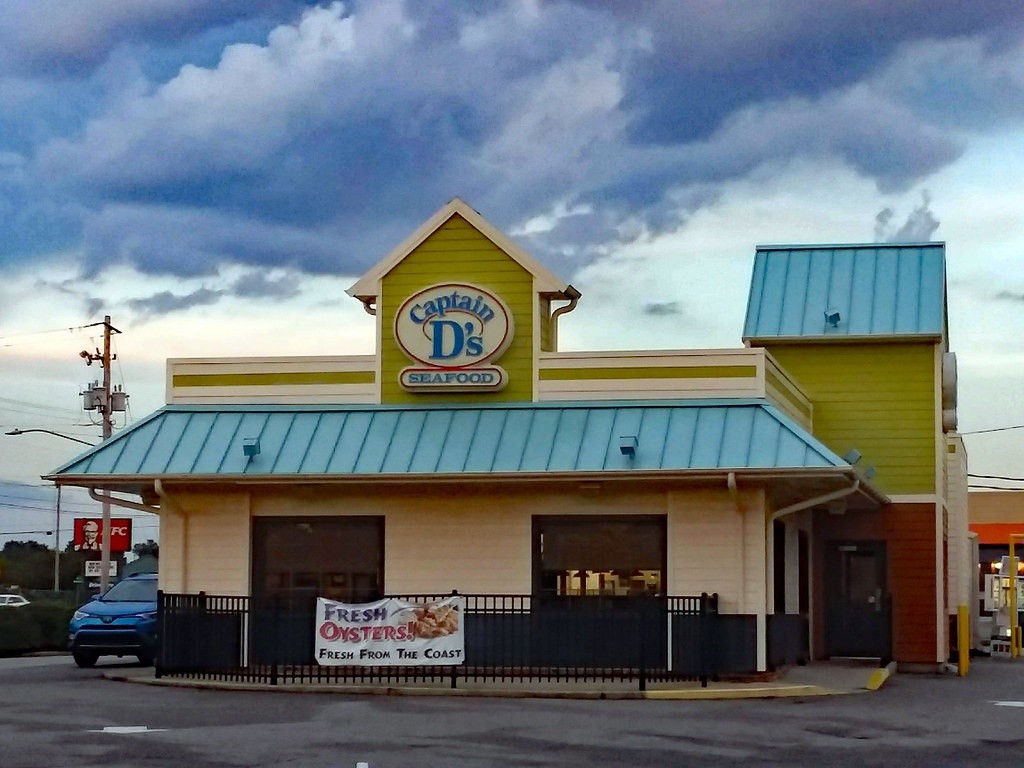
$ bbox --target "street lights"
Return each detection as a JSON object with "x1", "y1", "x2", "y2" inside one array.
[{"x1": 3, "y1": 428, "x2": 112, "y2": 593}]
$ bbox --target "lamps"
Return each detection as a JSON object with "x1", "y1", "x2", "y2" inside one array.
[
  {"x1": 843, "y1": 448, "x2": 863, "y2": 465},
  {"x1": 617, "y1": 435, "x2": 638, "y2": 459},
  {"x1": 242, "y1": 437, "x2": 261, "y2": 462},
  {"x1": 863, "y1": 465, "x2": 878, "y2": 479},
  {"x1": 823, "y1": 307, "x2": 842, "y2": 328}
]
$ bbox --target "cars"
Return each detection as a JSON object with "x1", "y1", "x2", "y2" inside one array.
[
  {"x1": 65, "y1": 571, "x2": 159, "y2": 668},
  {"x1": 0, "y1": 594, "x2": 31, "y2": 609}
]
